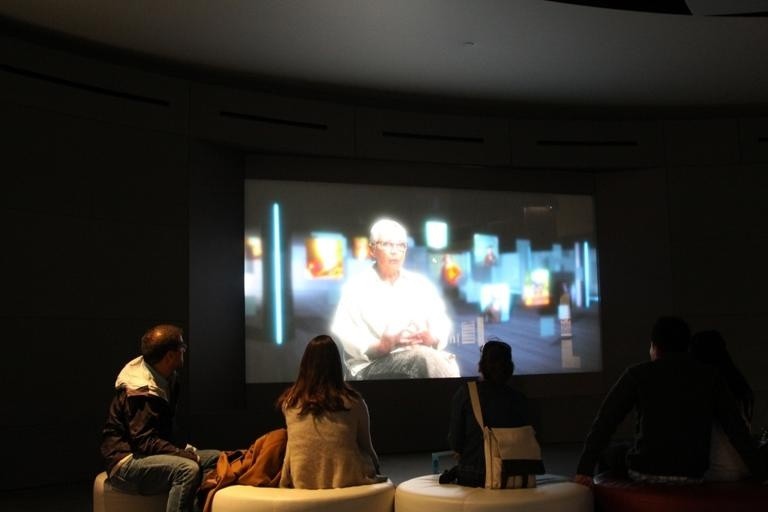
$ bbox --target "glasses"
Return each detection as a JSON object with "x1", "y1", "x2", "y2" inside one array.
[
  {"x1": 171, "y1": 344, "x2": 188, "y2": 352},
  {"x1": 374, "y1": 238, "x2": 407, "y2": 251}
]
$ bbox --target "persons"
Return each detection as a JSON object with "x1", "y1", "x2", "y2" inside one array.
[
  {"x1": 448, "y1": 343, "x2": 541, "y2": 489},
  {"x1": 695, "y1": 324, "x2": 757, "y2": 481},
  {"x1": 274, "y1": 333, "x2": 380, "y2": 491},
  {"x1": 574, "y1": 315, "x2": 717, "y2": 488},
  {"x1": 101, "y1": 325, "x2": 221, "y2": 512},
  {"x1": 330, "y1": 218, "x2": 461, "y2": 379}
]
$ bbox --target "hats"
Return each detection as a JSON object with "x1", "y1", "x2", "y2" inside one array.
[{"x1": 481, "y1": 341, "x2": 512, "y2": 363}]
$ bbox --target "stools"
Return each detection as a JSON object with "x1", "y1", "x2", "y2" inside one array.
[{"x1": 93, "y1": 472, "x2": 169, "y2": 512}]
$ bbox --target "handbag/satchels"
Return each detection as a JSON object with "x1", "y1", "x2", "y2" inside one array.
[{"x1": 468, "y1": 382, "x2": 547, "y2": 489}]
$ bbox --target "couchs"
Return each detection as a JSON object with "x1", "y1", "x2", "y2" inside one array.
[
  {"x1": 395, "y1": 472, "x2": 593, "y2": 512},
  {"x1": 210, "y1": 479, "x2": 396, "y2": 512},
  {"x1": 589, "y1": 454, "x2": 768, "y2": 512}
]
